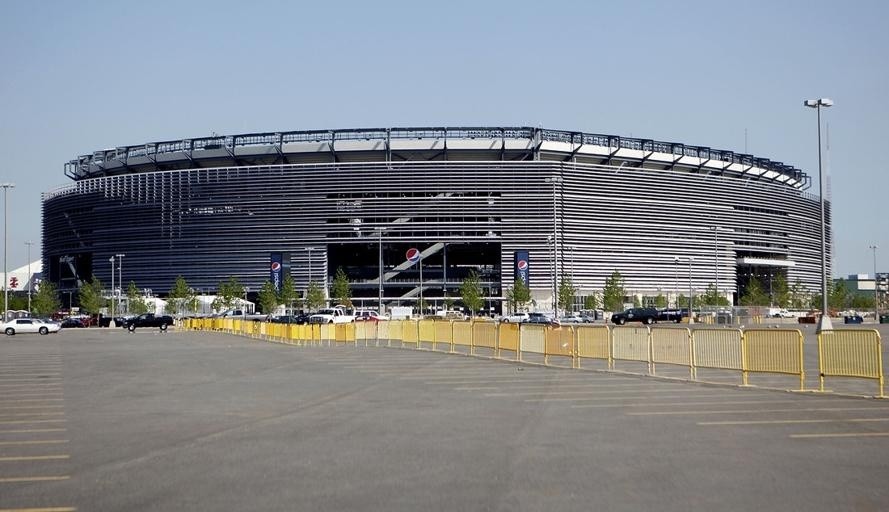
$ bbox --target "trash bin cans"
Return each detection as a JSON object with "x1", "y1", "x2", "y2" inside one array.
[
  {"x1": 297, "y1": 316, "x2": 306, "y2": 324},
  {"x1": 844, "y1": 316, "x2": 861, "y2": 324},
  {"x1": 879, "y1": 315, "x2": 889, "y2": 324}
]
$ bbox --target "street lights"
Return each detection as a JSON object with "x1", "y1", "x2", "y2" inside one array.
[
  {"x1": 419, "y1": 254, "x2": 424, "y2": 314},
  {"x1": 689, "y1": 256, "x2": 694, "y2": 325},
  {"x1": 0, "y1": 182, "x2": 16, "y2": 322},
  {"x1": 109, "y1": 255, "x2": 116, "y2": 329},
  {"x1": 116, "y1": 253, "x2": 124, "y2": 317},
  {"x1": 713, "y1": 224, "x2": 721, "y2": 326},
  {"x1": 804, "y1": 97, "x2": 834, "y2": 333},
  {"x1": 870, "y1": 245, "x2": 879, "y2": 322},
  {"x1": 674, "y1": 256, "x2": 680, "y2": 309},
  {"x1": 24, "y1": 239, "x2": 34, "y2": 312},
  {"x1": 305, "y1": 246, "x2": 317, "y2": 313},
  {"x1": 544, "y1": 176, "x2": 575, "y2": 318},
  {"x1": 375, "y1": 226, "x2": 387, "y2": 315}
]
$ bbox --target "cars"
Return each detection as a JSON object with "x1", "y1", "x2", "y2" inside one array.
[{"x1": 611, "y1": 306, "x2": 875, "y2": 326}]
